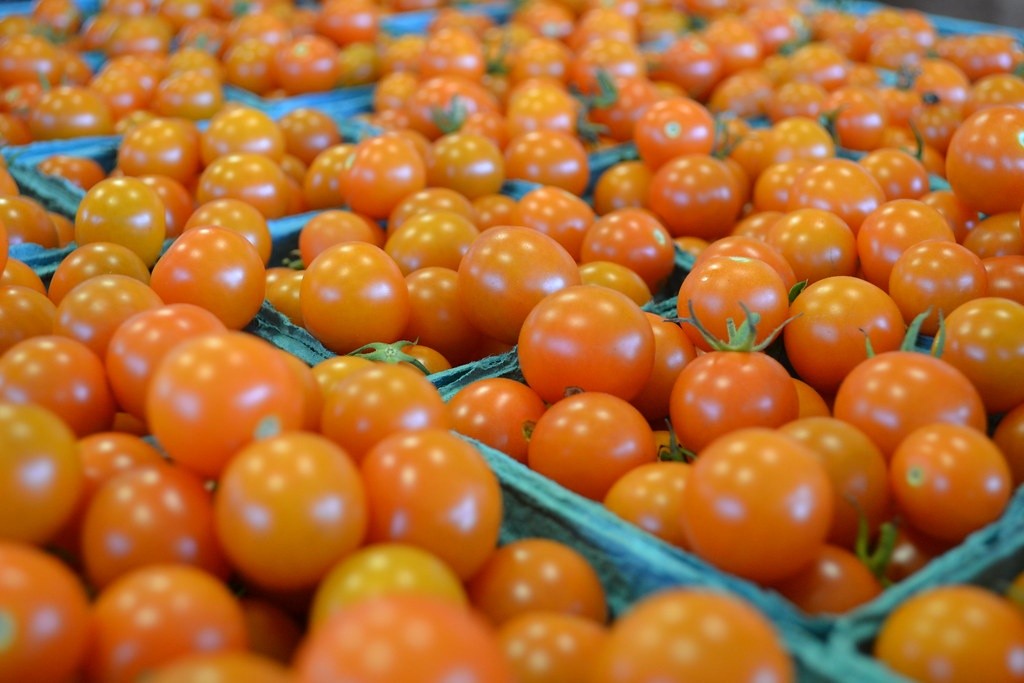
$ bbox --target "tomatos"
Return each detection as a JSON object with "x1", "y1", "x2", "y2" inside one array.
[{"x1": 0, "y1": 0, "x2": 1024, "y2": 683}]
